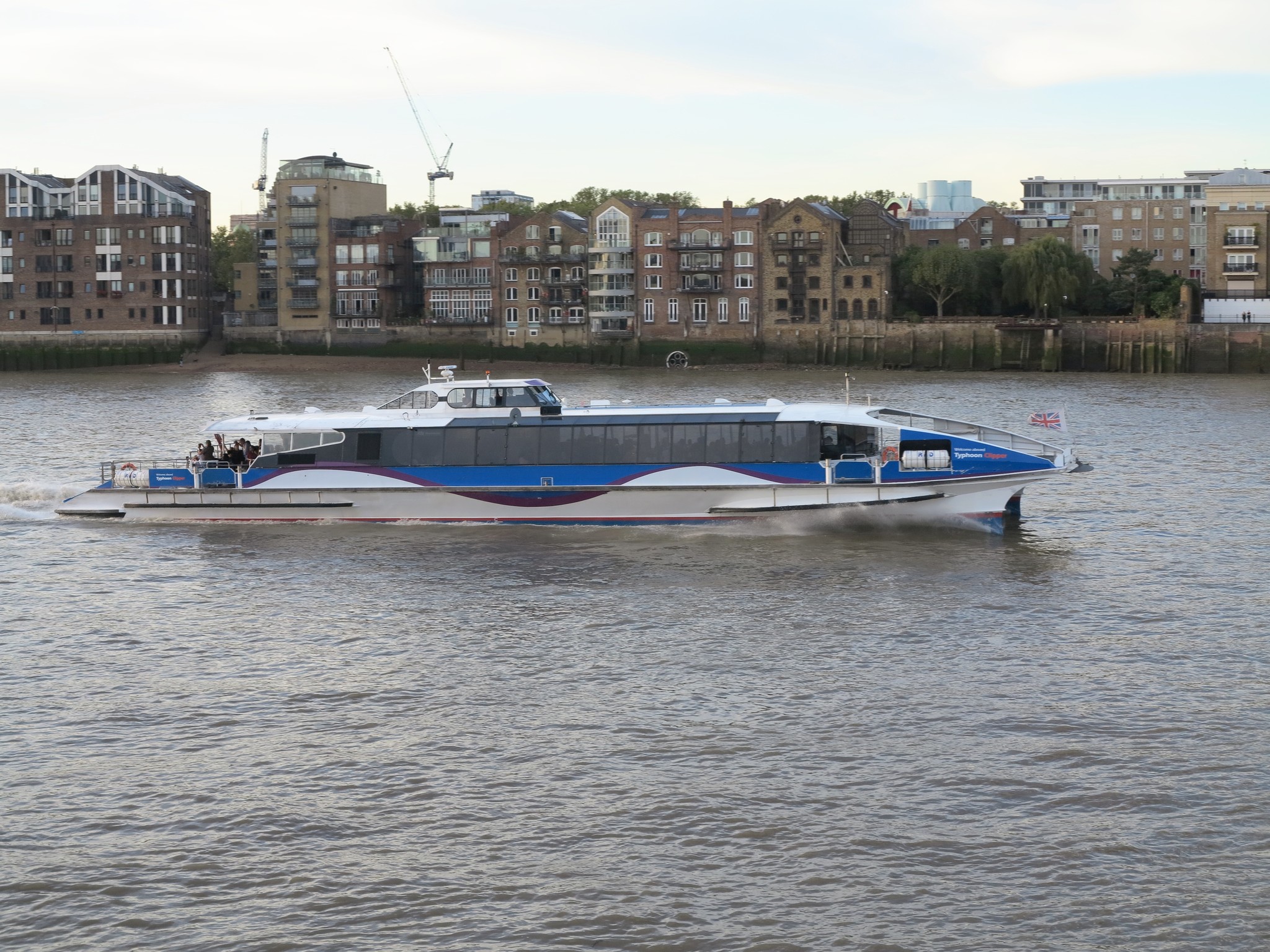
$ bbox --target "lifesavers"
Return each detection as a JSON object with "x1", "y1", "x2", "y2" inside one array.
[
  {"x1": 882, "y1": 446, "x2": 898, "y2": 464},
  {"x1": 120, "y1": 463, "x2": 137, "y2": 471}
]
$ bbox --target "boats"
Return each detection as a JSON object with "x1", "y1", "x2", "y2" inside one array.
[{"x1": 51, "y1": 358, "x2": 1092, "y2": 535}]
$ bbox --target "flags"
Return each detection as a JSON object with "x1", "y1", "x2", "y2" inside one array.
[{"x1": 1028, "y1": 406, "x2": 1070, "y2": 433}]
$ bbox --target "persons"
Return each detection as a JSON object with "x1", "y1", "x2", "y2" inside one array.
[
  {"x1": 507, "y1": 387, "x2": 515, "y2": 397},
  {"x1": 461, "y1": 397, "x2": 474, "y2": 408},
  {"x1": 487, "y1": 396, "x2": 495, "y2": 408},
  {"x1": 188, "y1": 437, "x2": 291, "y2": 474},
  {"x1": 559, "y1": 422, "x2": 856, "y2": 466}
]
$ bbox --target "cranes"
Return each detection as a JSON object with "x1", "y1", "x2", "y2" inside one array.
[
  {"x1": 251, "y1": 128, "x2": 270, "y2": 221},
  {"x1": 384, "y1": 46, "x2": 455, "y2": 205}
]
